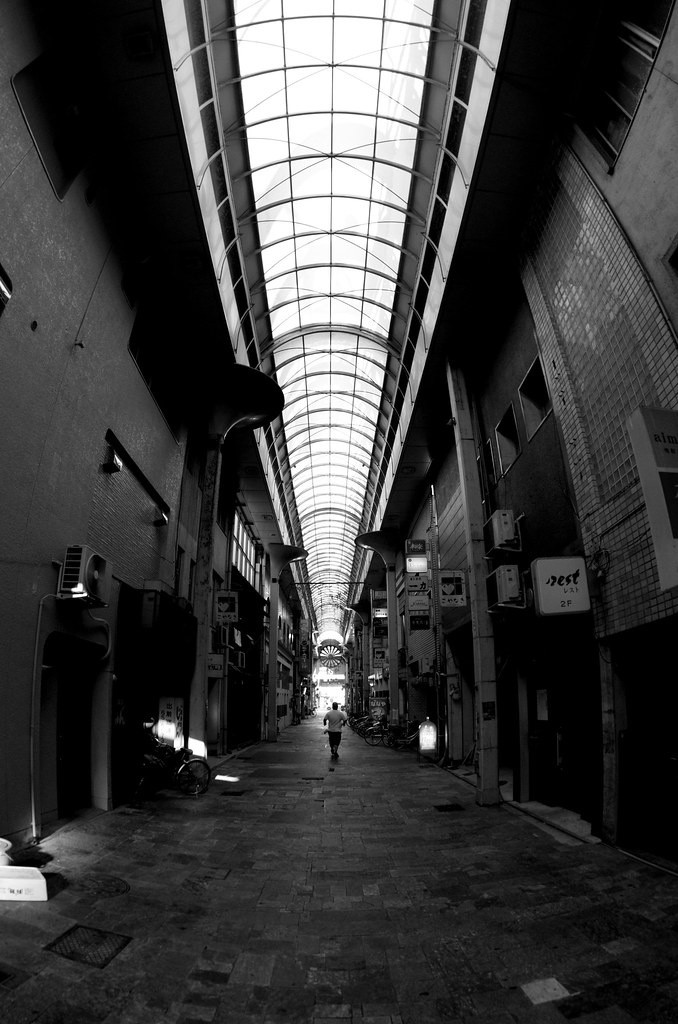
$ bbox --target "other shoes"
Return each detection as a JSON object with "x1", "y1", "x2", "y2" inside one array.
[{"x1": 331, "y1": 752, "x2": 340, "y2": 759}]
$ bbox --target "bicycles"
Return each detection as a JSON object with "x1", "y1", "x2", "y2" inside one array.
[
  {"x1": 133, "y1": 734, "x2": 212, "y2": 807},
  {"x1": 305, "y1": 706, "x2": 317, "y2": 716},
  {"x1": 347, "y1": 711, "x2": 420, "y2": 749}
]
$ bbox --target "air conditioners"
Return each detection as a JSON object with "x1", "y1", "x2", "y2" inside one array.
[
  {"x1": 482, "y1": 509, "x2": 522, "y2": 559},
  {"x1": 485, "y1": 564, "x2": 527, "y2": 615},
  {"x1": 56, "y1": 545, "x2": 113, "y2": 612}
]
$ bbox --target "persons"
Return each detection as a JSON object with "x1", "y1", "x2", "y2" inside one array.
[{"x1": 323, "y1": 702, "x2": 347, "y2": 761}]
